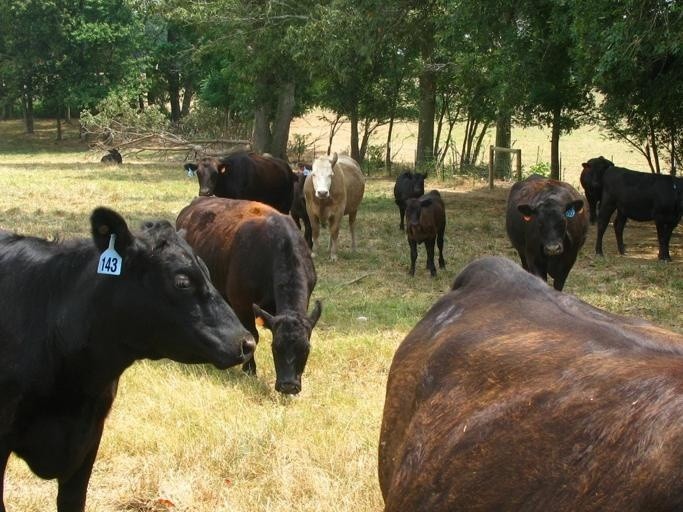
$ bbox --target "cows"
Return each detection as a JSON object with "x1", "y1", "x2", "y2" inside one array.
[
  {"x1": 196, "y1": 171, "x2": 197, "y2": 172},
  {"x1": 393, "y1": 188, "x2": 448, "y2": 278},
  {"x1": 100, "y1": 147, "x2": 122, "y2": 165},
  {"x1": 183, "y1": 150, "x2": 366, "y2": 263},
  {"x1": 391, "y1": 168, "x2": 430, "y2": 231},
  {"x1": 175, "y1": 196, "x2": 323, "y2": 394},
  {"x1": 373, "y1": 252, "x2": 683, "y2": 512},
  {"x1": 0, "y1": 202, "x2": 258, "y2": 512},
  {"x1": 578, "y1": 155, "x2": 616, "y2": 222},
  {"x1": 504, "y1": 170, "x2": 590, "y2": 293},
  {"x1": 593, "y1": 165, "x2": 683, "y2": 263}
]
